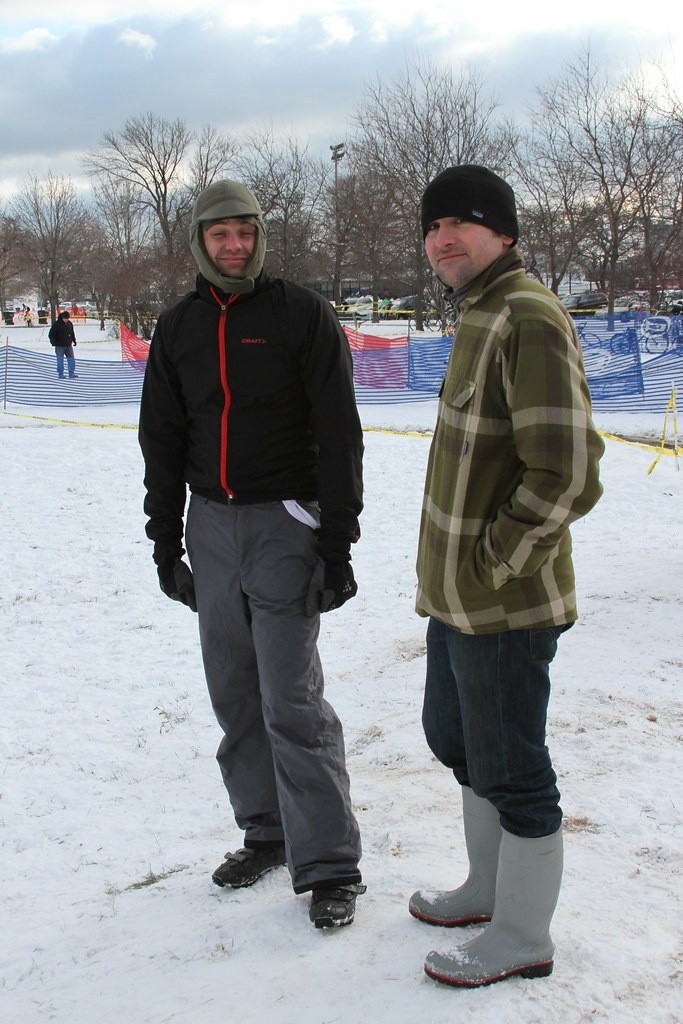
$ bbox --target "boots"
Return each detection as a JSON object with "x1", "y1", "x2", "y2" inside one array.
[
  {"x1": 425, "y1": 818, "x2": 564, "y2": 988},
  {"x1": 409, "y1": 785, "x2": 502, "y2": 925}
]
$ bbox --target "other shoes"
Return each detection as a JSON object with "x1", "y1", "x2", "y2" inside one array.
[
  {"x1": 69, "y1": 372, "x2": 78, "y2": 378},
  {"x1": 58, "y1": 373, "x2": 66, "y2": 379}
]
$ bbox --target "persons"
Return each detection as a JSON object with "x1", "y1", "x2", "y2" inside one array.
[
  {"x1": 49, "y1": 311, "x2": 77, "y2": 379},
  {"x1": 411, "y1": 165, "x2": 606, "y2": 990},
  {"x1": 138, "y1": 182, "x2": 364, "y2": 926}
]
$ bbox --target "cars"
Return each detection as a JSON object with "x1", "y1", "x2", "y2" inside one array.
[
  {"x1": 334, "y1": 297, "x2": 425, "y2": 320},
  {"x1": 560, "y1": 290, "x2": 683, "y2": 319}
]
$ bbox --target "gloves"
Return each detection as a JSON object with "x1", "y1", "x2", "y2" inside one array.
[
  {"x1": 305, "y1": 540, "x2": 359, "y2": 617},
  {"x1": 73, "y1": 340, "x2": 76, "y2": 346},
  {"x1": 151, "y1": 547, "x2": 197, "y2": 612}
]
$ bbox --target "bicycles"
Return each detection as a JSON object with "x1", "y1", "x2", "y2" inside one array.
[
  {"x1": 409, "y1": 309, "x2": 442, "y2": 332},
  {"x1": 573, "y1": 317, "x2": 683, "y2": 355}
]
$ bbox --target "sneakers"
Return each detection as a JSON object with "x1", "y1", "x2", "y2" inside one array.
[
  {"x1": 307, "y1": 881, "x2": 368, "y2": 929},
  {"x1": 212, "y1": 839, "x2": 287, "y2": 889}
]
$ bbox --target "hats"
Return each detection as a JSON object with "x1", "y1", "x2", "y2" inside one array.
[
  {"x1": 189, "y1": 179, "x2": 265, "y2": 295},
  {"x1": 420, "y1": 164, "x2": 519, "y2": 248},
  {"x1": 62, "y1": 311, "x2": 70, "y2": 319}
]
